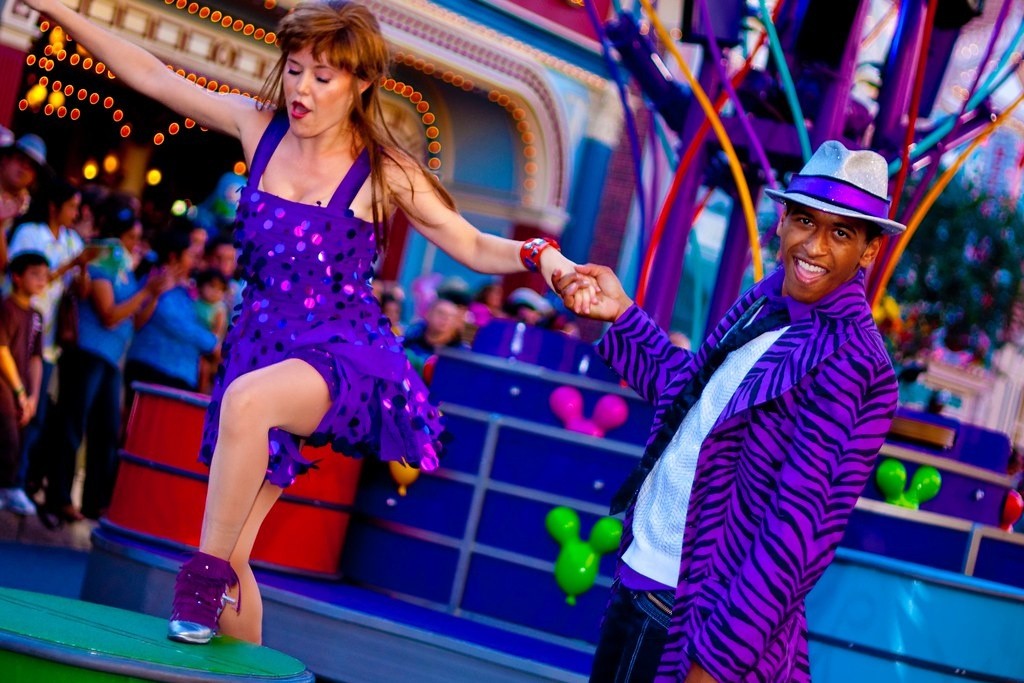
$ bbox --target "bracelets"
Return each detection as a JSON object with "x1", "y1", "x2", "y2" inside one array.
[{"x1": 520, "y1": 236, "x2": 561, "y2": 273}]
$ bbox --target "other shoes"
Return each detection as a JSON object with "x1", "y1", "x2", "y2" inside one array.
[
  {"x1": 65, "y1": 504, "x2": 83, "y2": 521},
  {"x1": 79, "y1": 503, "x2": 109, "y2": 520},
  {"x1": 0, "y1": 488, "x2": 35, "y2": 516},
  {"x1": 39, "y1": 503, "x2": 64, "y2": 531}
]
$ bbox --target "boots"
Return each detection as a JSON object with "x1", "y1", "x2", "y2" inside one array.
[{"x1": 168, "y1": 551, "x2": 242, "y2": 645}]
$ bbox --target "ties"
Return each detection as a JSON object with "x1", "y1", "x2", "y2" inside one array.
[{"x1": 609, "y1": 294, "x2": 790, "y2": 516}]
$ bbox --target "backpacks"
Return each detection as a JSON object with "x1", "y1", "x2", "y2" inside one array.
[{"x1": 54, "y1": 281, "x2": 78, "y2": 348}]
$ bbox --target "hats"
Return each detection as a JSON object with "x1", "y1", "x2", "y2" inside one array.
[
  {"x1": 763, "y1": 140, "x2": 907, "y2": 236},
  {"x1": 17, "y1": 133, "x2": 45, "y2": 165}
]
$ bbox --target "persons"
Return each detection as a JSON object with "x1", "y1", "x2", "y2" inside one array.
[
  {"x1": 21, "y1": 0, "x2": 601, "y2": 644},
  {"x1": 0, "y1": 136, "x2": 580, "y2": 529},
  {"x1": 552, "y1": 137, "x2": 910, "y2": 683}
]
